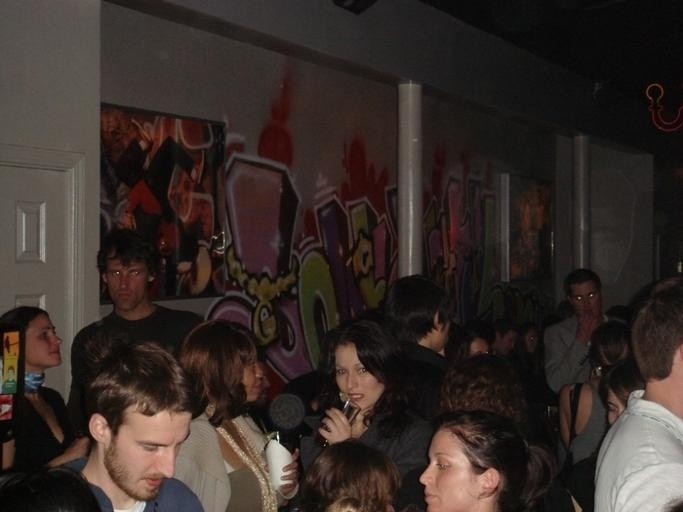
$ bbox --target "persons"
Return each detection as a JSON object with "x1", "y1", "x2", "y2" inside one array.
[
  {"x1": 593, "y1": 276, "x2": 682, "y2": 512},
  {"x1": 62, "y1": 223, "x2": 204, "y2": 432},
  {"x1": 0, "y1": 267, "x2": 646, "y2": 512}
]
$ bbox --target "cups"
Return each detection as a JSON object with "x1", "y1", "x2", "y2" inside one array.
[
  {"x1": 315, "y1": 393, "x2": 363, "y2": 448},
  {"x1": 264, "y1": 438, "x2": 296, "y2": 491}
]
